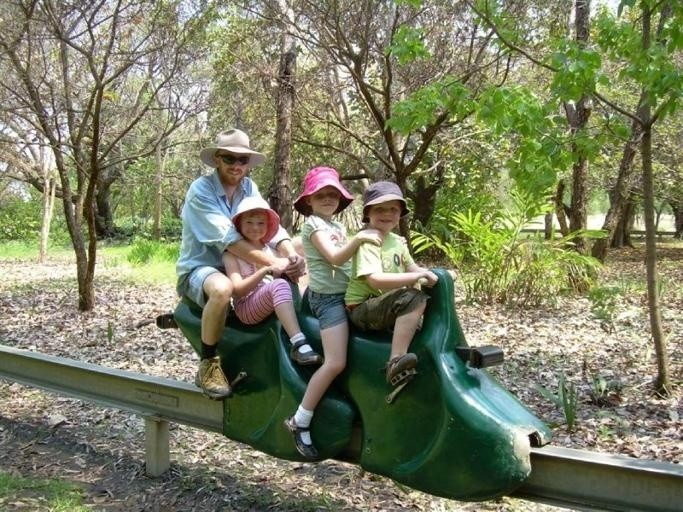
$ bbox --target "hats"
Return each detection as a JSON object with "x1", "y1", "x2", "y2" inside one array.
[
  {"x1": 362, "y1": 181, "x2": 410, "y2": 223},
  {"x1": 292, "y1": 166, "x2": 355, "y2": 215},
  {"x1": 198, "y1": 128, "x2": 266, "y2": 169},
  {"x1": 230, "y1": 196, "x2": 279, "y2": 243}
]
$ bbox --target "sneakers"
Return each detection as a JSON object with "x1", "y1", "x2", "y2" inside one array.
[
  {"x1": 385, "y1": 352, "x2": 418, "y2": 382},
  {"x1": 195, "y1": 354, "x2": 234, "y2": 400}
]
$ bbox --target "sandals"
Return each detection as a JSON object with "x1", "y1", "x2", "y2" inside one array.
[
  {"x1": 289, "y1": 339, "x2": 323, "y2": 364},
  {"x1": 283, "y1": 415, "x2": 319, "y2": 460}
]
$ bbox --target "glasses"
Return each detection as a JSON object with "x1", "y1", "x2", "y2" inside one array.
[{"x1": 215, "y1": 154, "x2": 250, "y2": 165}]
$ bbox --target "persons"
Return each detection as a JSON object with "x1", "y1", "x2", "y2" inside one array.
[
  {"x1": 343, "y1": 181, "x2": 457, "y2": 383},
  {"x1": 284, "y1": 166, "x2": 384, "y2": 460},
  {"x1": 175, "y1": 128, "x2": 306, "y2": 401},
  {"x1": 222, "y1": 197, "x2": 322, "y2": 366}
]
